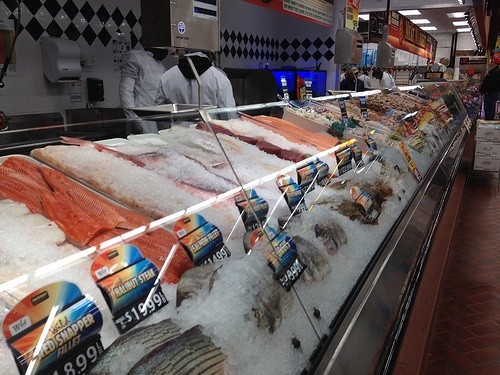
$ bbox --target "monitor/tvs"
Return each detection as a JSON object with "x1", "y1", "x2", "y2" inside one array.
[
  {"x1": 358, "y1": 14, "x2": 370, "y2": 34},
  {"x1": 297, "y1": 69, "x2": 327, "y2": 96},
  {"x1": 272, "y1": 68, "x2": 296, "y2": 94}
]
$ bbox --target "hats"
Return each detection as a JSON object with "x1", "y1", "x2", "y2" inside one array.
[
  {"x1": 494, "y1": 52, "x2": 500, "y2": 64},
  {"x1": 439, "y1": 57, "x2": 444, "y2": 63},
  {"x1": 349, "y1": 67, "x2": 359, "y2": 74}
]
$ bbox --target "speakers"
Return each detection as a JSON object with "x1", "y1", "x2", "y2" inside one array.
[{"x1": 87, "y1": 78, "x2": 104, "y2": 101}]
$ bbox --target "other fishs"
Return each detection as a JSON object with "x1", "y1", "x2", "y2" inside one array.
[{"x1": 84, "y1": 156, "x2": 405, "y2": 375}]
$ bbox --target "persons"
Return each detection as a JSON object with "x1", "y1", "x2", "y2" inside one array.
[
  {"x1": 340, "y1": 68, "x2": 348, "y2": 82},
  {"x1": 340, "y1": 67, "x2": 364, "y2": 92},
  {"x1": 119, "y1": 48, "x2": 169, "y2": 139},
  {"x1": 478, "y1": 59, "x2": 500, "y2": 120},
  {"x1": 358, "y1": 72, "x2": 374, "y2": 90},
  {"x1": 372, "y1": 68, "x2": 395, "y2": 89},
  {"x1": 154, "y1": 51, "x2": 239, "y2": 131}
]
